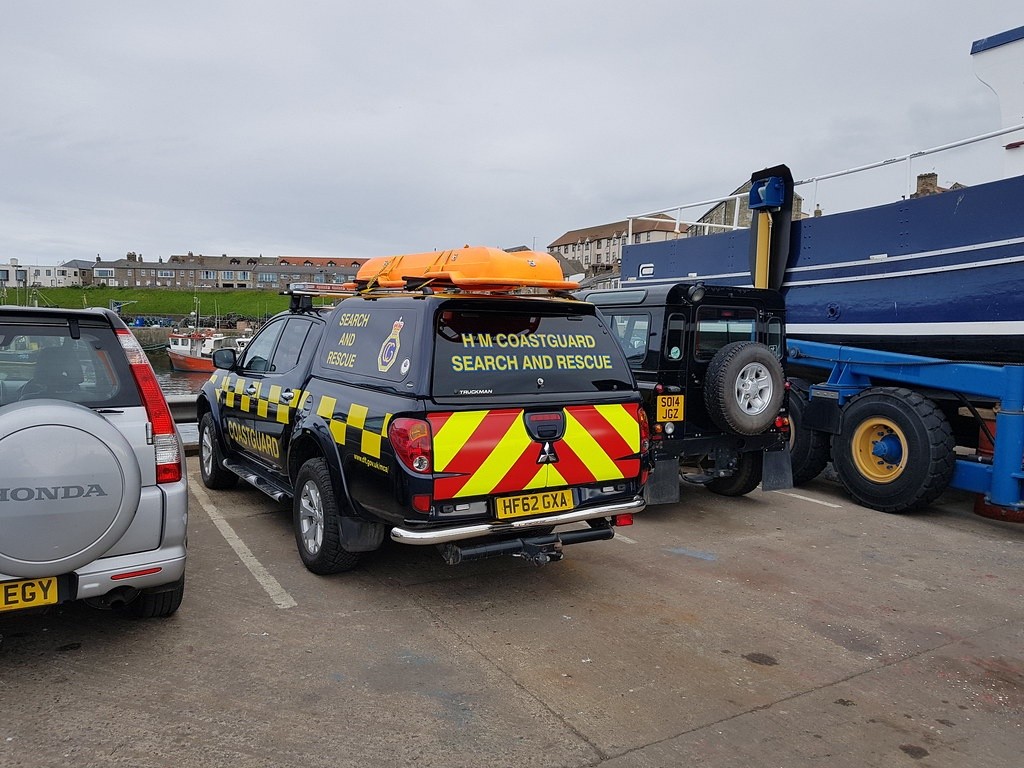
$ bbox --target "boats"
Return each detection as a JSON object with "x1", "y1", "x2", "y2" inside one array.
[{"x1": 167, "y1": 322, "x2": 257, "y2": 375}]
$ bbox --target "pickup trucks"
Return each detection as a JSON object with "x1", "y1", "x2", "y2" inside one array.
[{"x1": 199, "y1": 246, "x2": 654, "y2": 578}]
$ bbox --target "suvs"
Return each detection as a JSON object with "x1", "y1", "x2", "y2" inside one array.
[
  {"x1": 0, "y1": 302, "x2": 189, "y2": 618},
  {"x1": 551, "y1": 276, "x2": 794, "y2": 509}
]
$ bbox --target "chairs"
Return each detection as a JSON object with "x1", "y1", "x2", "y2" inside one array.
[{"x1": 17, "y1": 346, "x2": 94, "y2": 403}]
